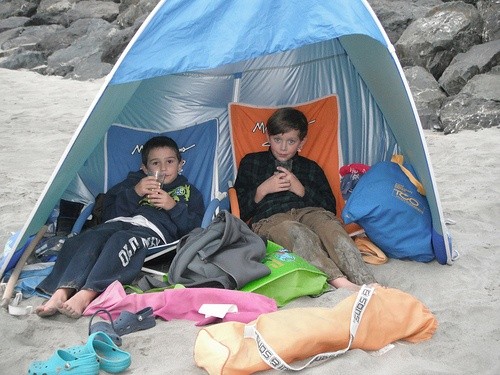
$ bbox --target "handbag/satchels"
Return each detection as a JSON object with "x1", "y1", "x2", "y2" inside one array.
[
  {"x1": 341, "y1": 154, "x2": 436, "y2": 263},
  {"x1": 238, "y1": 240, "x2": 329, "y2": 306},
  {"x1": 193, "y1": 281, "x2": 438, "y2": 374}
]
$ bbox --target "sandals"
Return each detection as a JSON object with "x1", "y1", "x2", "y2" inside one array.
[
  {"x1": 111, "y1": 307, "x2": 156, "y2": 335},
  {"x1": 88, "y1": 309, "x2": 123, "y2": 346},
  {"x1": 27, "y1": 350, "x2": 100, "y2": 375},
  {"x1": 66, "y1": 331, "x2": 132, "y2": 374}
]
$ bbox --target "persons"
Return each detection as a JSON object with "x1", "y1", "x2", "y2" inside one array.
[
  {"x1": 34, "y1": 137, "x2": 204, "y2": 318},
  {"x1": 232, "y1": 106, "x2": 375, "y2": 288}
]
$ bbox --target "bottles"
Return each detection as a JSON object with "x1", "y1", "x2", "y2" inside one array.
[{"x1": 26, "y1": 232, "x2": 78, "y2": 265}]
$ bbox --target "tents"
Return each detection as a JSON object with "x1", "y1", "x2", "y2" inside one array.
[{"x1": 1, "y1": 0, "x2": 460, "y2": 297}]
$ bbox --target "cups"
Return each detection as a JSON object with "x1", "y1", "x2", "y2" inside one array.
[
  {"x1": 275, "y1": 158, "x2": 293, "y2": 172},
  {"x1": 147, "y1": 167, "x2": 167, "y2": 205}
]
